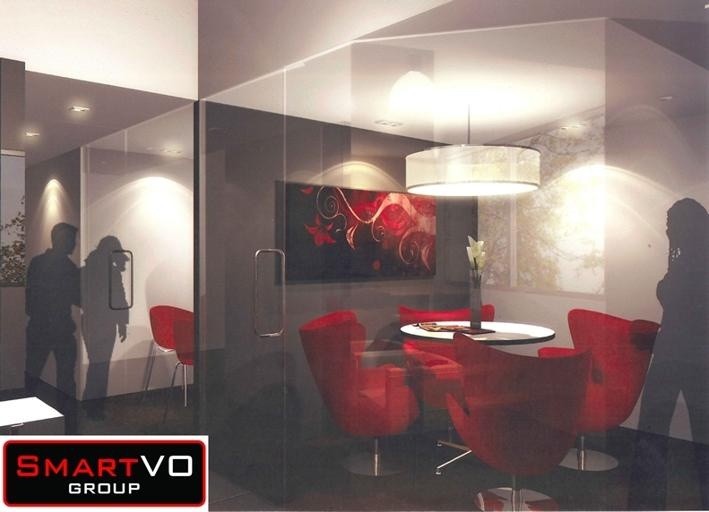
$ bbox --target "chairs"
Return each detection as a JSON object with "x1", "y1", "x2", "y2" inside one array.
[
  {"x1": 139, "y1": 302, "x2": 195, "y2": 427},
  {"x1": 300, "y1": 299, "x2": 662, "y2": 511}
]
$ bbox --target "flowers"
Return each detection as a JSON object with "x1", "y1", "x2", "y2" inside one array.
[{"x1": 464, "y1": 234, "x2": 486, "y2": 289}]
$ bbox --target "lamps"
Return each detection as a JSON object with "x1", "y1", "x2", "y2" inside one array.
[{"x1": 403, "y1": 104, "x2": 541, "y2": 199}]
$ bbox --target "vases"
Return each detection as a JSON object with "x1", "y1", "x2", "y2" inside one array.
[{"x1": 467, "y1": 270, "x2": 483, "y2": 330}]
